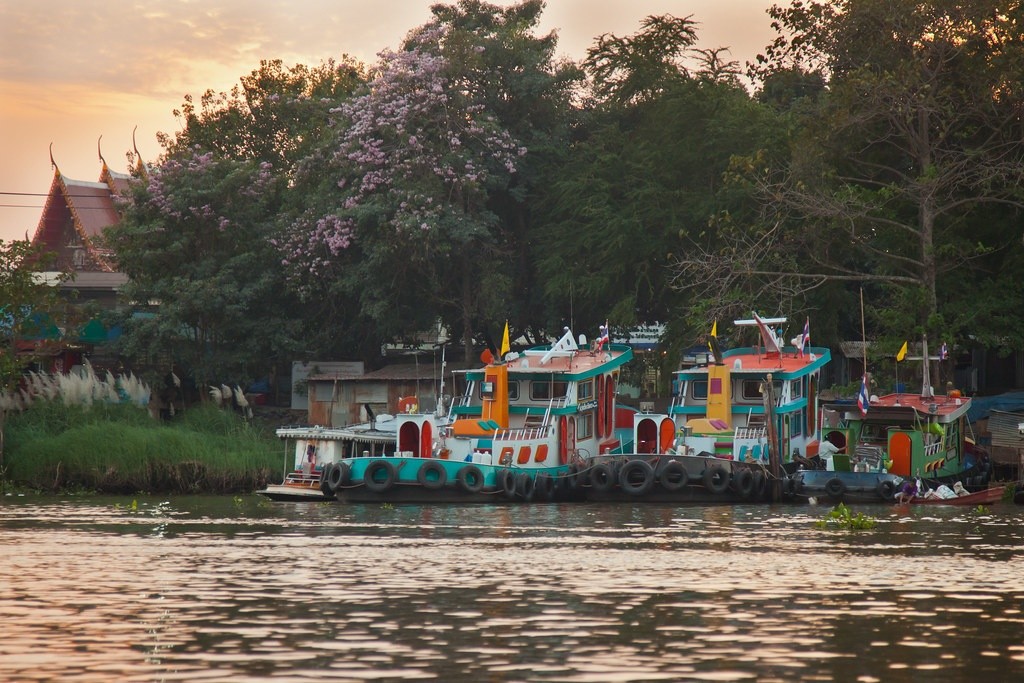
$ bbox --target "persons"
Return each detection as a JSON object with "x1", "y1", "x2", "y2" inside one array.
[
  {"x1": 819, "y1": 435, "x2": 848, "y2": 471},
  {"x1": 894, "y1": 478, "x2": 919, "y2": 506}
]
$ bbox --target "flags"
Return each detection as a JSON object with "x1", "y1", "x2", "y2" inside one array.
[
  {"x1": 501, "y1": 324, "x2": 510, "y2": 356},
  {"x1": 858, "y1": 374, "x2": 869, "y2": 416},
  {"x1": 897, "y1": 341, "x2": 907, "y2": 361},
  {"x1": 800, "y1": 320, "x2": 809, "y2": 358},
  {"x1": 940, "y1": 345, "x2": 948, "y2": 360},
  {"x1": 708, "y1": 322, "x2": 715, "y2": 351},
  {"x1": 598, "y1": 323, "x2": 608, "y2": 355}
]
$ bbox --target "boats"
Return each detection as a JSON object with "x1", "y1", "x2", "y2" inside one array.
[
  {"x1": 254, "y1": 426, "x2": 360, "y2": 500},
  {"x1": 320, "y1": 321, "x2": 641, "y2": 503},
  {"x1": 567, "y1": 310, "x2": 831, "y2": 499},
  {"x1": 906, "y1": 487, "x2": 1005, "y2": 508},
  {"x1": 785, "y1": 332, "x2": 990, "y2": 501}
]
